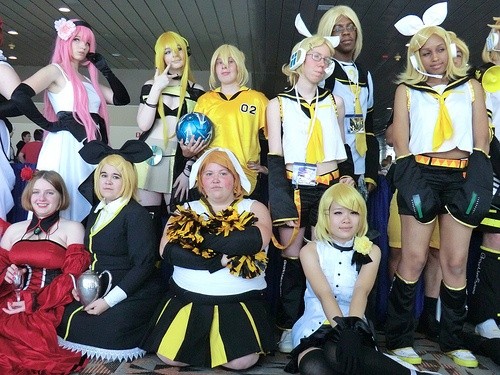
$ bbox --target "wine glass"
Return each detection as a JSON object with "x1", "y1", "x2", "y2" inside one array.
[{"x1": 11, "y1": 268, "x2": 24, "y2": 309}]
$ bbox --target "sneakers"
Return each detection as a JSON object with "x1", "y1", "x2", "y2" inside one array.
[
  {"x1": 387, "y1": 347, "x2": 421, "y2": 365},
  {"x1": 444, "y1": 349, "x2": 479, "y2": 368}
]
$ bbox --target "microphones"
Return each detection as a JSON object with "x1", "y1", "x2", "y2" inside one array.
[{"x1": 410, "y1": 56, "x2": 443, "y2": 79}]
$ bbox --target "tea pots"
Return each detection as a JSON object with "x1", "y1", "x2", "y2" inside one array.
[{"x1": 68, "y1": 265, "x2": 113, "y2": 311}]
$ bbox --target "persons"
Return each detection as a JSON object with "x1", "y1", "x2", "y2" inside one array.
[
  {"x1": 373, "y1": 31, "x2": 469, "y2": 336},
  {"x1": 55, "y1": 154, "x2": 161, "y2": 359},
  {"x1": 319, "y1": 5, "x2": 381, "y2": 216},
  {"x1": 288, "y1": 183, "x2": 442, "y2": 375},
  {"x1": 16, "y1": 131, "x2": 31, "y2": 160},
  {"x1": 386, "y1": 25, "x2": 479, "y2": 369},
  {"x1": 264, "y1": 36, "x2": 345, "y2": 352},
  {"x1": 0, "y1": 167, "x2": 90, "y2": 375},
  {"x1": 154, "y1": 148, "x2": 274, "y2": 369},
  {"x1": 474, "y1": 17, "x2": 500, "y2": 338},
  {"x1": 175, "y1": 43, "x2": 271, "y2": 206},
  {"x1": 380, "y1": 155, "x2": 394, "y2": 174},
  {"x1": 18, "y1": 129, "x2": 45, "y2": 164},
  {"x1": 131, "y1": 30, "x2": 205, "y2": 279},
  {"x1": 0, "y1": 20, "x2": 26, "y2": 239},
  {"x1": 11, "y1": 19, "x2": 131, "y2": 228}
]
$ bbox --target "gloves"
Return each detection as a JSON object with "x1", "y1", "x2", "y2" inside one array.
[
  {"x1": 86, "y1": 53, "x2": 130, "y2": 106},
  {"x1": 323, "y1": 316, "x2": 376, "y2": 375},
  {"x1": 11, "y1": 83, "x2": 78, "y2": 132}
]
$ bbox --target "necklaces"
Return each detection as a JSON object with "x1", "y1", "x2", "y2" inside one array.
[{"x1": 334, "y1": 55, "x2": 366, "y2": 133}]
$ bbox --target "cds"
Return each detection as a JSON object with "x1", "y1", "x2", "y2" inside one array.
[{"x1": 144, "y1": 145, "x2": 162, "y2": 166}]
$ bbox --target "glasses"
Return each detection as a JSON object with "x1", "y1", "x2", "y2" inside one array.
[
  {"x1": 332, "y1": 24, "x2": 357, "y2": 35},
  {"x1": 306, "y1": 52, "x2": 332, "y2": 65}
]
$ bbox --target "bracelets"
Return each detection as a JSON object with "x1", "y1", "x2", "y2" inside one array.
[
  {"x1": 144, "y1": 99, "x2": 158, "y2": 108},
  {"x1": 183, "y1": 158, "x2": 197, "y2": 178}
]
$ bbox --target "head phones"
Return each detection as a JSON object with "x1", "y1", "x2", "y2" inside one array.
[
  {"x1": 485, "y1": 28, "x2": 499, "y2": 51},
  {"x1": 289, "y1": 38, "x2": 335, "y2": 82},
  {"x1": 182, "y1": 36, "x2": 191, "y2": 56}
]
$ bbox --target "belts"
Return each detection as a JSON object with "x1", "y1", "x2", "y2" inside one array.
[
  {"x1": 414, "y1": 154, "x2": 468, "y2": 169},
  {"x1": 286, "y1": 169, "x2": 339, "y2": 185}
]
